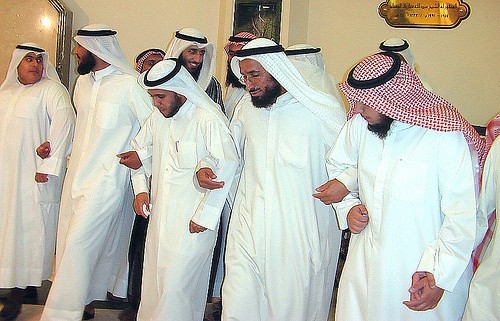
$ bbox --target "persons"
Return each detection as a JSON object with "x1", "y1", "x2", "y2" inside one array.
[
  {"x1": 193, "y1": 39, "x2": 359, "y2": 321},
  {"x1": 134, "y1": 47, "x2": 166, "y2": 74},
  {"x1": 118, "y1": 27, "x2": 227, "y2": 321},
  {"x1": 129, "y1": 58, "x2": 242, "y2": 321},
  {"x1": 211, "y1": 32, "x2": 257, "y2": 321},
  {"x1": 284, "y1": 44, "x2": 346, "y2": 118},
  {"x1": 36, "y1": 24, "x2": 153, "y2": 321},
  {"x1": 325, "y1": 51, "x2": 485, "y2": 321},
  {"x1": 1, "y1": 42, "x2": 77, "y2": 321},
  {"x1": 411, "y1": 113, "x2": 500, "y2": 321}
]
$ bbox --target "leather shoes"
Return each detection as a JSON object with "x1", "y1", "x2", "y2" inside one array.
[{"x1": 0, "y1": 293, "x2": 40, "y2": 321}]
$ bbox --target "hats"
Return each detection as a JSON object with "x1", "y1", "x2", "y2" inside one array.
[
  {"x1": 0, "y1": 42, "x2": 77, "y2": 119},
  {"x1": 338, "y1": 51, "x2": 484, "y2": 189},
  {"x1": 379, "y1": 38, "x2": 432, "y2": 92},
  {"x1": 133, "y1": 27, "x2": 347, "y2": 161},
  {"x1": 73, "y1": 23, "x2": 143, "y2": 81}
]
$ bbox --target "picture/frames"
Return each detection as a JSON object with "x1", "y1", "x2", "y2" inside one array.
[{"x1": 233, "y1": 0, "x2": 282, "y2": 45}]
